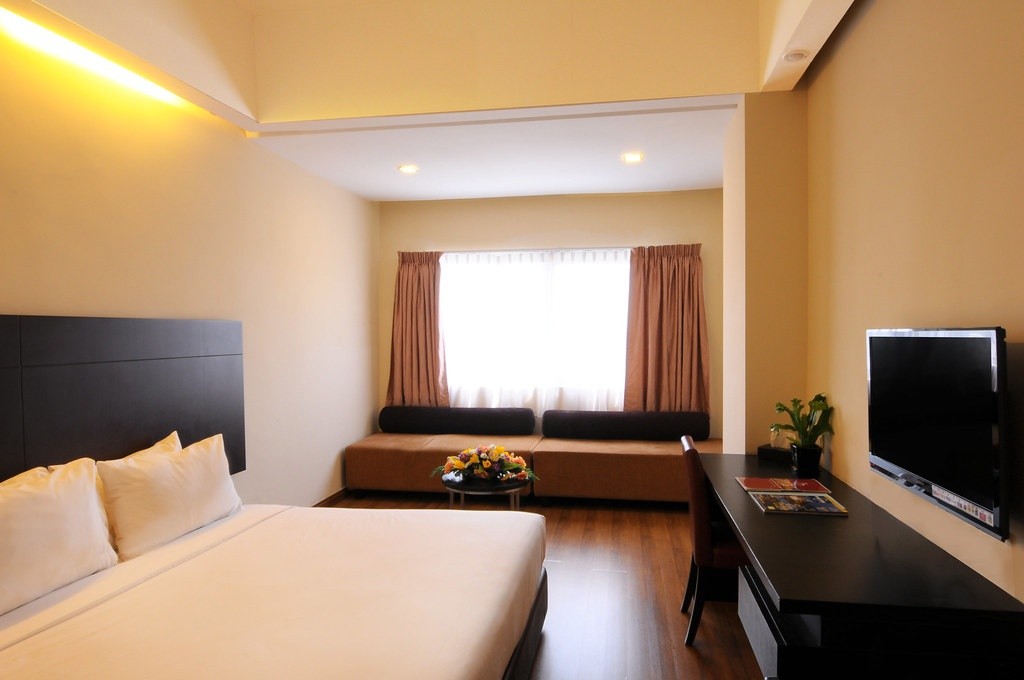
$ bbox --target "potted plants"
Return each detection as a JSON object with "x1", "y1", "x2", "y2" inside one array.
[{"x1": 771, "y1": 391, "x2": 835, "y2": 480}]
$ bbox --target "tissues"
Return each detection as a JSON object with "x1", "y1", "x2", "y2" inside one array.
[{"x1": 756, "y1": 428, "x2": 801, "y2": 466}]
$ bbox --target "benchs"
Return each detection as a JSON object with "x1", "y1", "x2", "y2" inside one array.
[
  {"x1": 344, "y1": 431, "x2": 544, "y2": 497},
  {"x1": 532, "y1": 437, "x2": 723, "y2": 503}
]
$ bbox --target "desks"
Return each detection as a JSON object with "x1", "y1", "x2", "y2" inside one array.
[{"x1": 696, "y1": 453, "x2": 1024, "y2": 680}]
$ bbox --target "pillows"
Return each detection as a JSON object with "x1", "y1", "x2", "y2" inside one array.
[
  {"x1": 542, "y1": 410, "x2": 711, "y2": 441},
  {"x1": 377, "y1": 404, "x2": 536, "y2": 435},
  {"x1": 96, "y1": 433, "x2": 244, "y2": 563},
  {"x1": 0, "y1": 457, "x2": 118, "y2": 617},
  {"x1": 48, "y1": 430, "x2": 183, "y2": 552}
]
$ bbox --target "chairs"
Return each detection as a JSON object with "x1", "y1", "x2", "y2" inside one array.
[{"x1": 680, "y1": 435, "x2": 749, "y2": 646}]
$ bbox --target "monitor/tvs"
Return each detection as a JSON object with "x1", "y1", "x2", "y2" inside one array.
[{"x1": 867, "y1": 325, "x2": 1010, "y2": 542}]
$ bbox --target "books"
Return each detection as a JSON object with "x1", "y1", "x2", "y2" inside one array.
[{"x1": 734, "y1": 476, "x2": 850, "y2": 517}]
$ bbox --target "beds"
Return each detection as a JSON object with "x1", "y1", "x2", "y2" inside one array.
[{"x1": 0, "y1": 504, "x2": 548, "y2": 680}]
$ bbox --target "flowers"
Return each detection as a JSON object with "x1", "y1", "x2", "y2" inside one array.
[{"x1": 431, "y1": 444, "x2": 540, "y2": 482}]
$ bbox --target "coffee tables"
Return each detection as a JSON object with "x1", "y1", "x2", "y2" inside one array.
[{"x1": 441, "y1": 471, "x2": 528, "y2": 511}]
